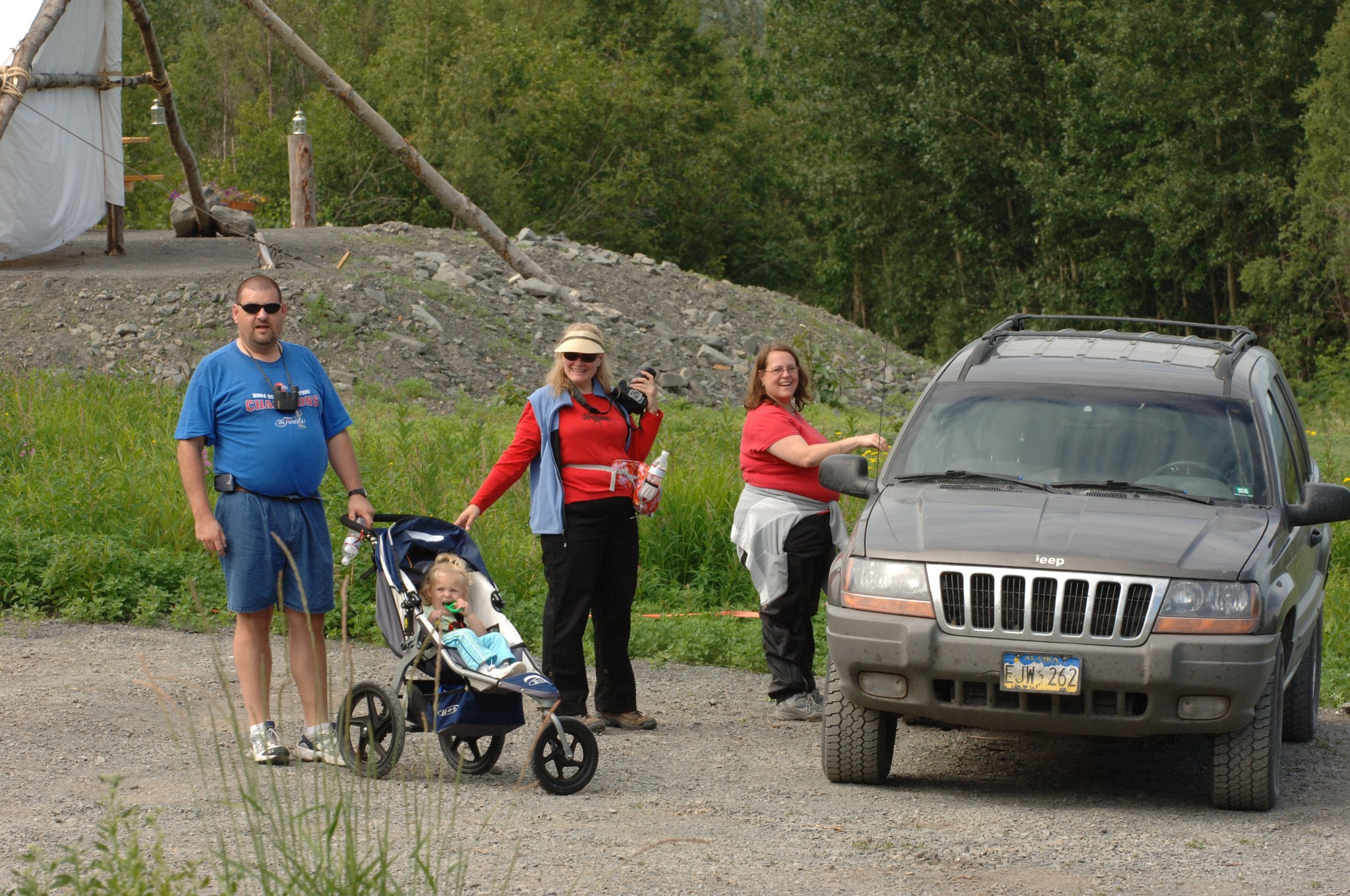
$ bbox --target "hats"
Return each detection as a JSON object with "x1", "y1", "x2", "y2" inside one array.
[{"x1": 554, "y1": 330, "x2": 605, "y2": 354}]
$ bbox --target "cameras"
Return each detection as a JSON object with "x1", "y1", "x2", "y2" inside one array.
[{"x1": 612, "y1": 365, "x2": 657, "y2": 415}]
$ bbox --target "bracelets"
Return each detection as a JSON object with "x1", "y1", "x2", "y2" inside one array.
[{"x1": 837, "y1": 441, "x2": 841, "y2": 455}]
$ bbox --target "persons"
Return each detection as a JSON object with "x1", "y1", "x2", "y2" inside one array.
[
  {"x1": 731, "y1": 343, "x2": 890, "y2": 722},
  {"x1": 453, "y1": 321, "x2": 664, "y2": 732},
  {"x1": 419, "y1": 551, "x2": 526, "y2": 695},
  {"x1": 171, "y1": 275, "x2": 376, "y2": 766}
]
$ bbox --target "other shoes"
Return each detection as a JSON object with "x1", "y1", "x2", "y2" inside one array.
[
  {"x1": 505, "y1": 662, "x2": 527, "y2": 676},
  {"x1": 483, "y1": 659, "x2": 514, "y2": 678}
]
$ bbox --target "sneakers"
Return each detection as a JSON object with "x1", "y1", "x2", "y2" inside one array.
[
  {"x1": 595, "y1": 705, "x2": 657, "y2": 731},
  {"x1": 573, "y1": 713, "x2": 606, "y2": 733},
  {"x1": 246, "y1": 720, "x2": 290, "y2": 765},
  {"x1": 292, "y1": 723, "x2": 368, "y2": 765},
  {"x1": 774, "y1": 693, "x2": 823, "y2": 722},
  {"x1": 808, "y1": 690, "x2": 825, "y2": 706}
]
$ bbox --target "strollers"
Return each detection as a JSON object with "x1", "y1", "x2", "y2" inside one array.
[{"x1": 336, "y1": 512, "x2": 603, "y2": 796}]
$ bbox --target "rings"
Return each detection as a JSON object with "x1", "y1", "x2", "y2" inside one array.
[
  {"x1": 645, "y1": 385, "x2": 649, "y2": 389},
  {"x1": 647, "y1": 381, "x2": 650, "y2": 384},
  {"x1": 878, "y1": 439, "x2": 881, "y2": 443}
]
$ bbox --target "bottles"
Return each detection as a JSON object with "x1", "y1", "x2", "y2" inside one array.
[
  {"x1": 341, "y1": 517, "x2": 367, "y2": 566},
  {"x1": 646, "y1": 450, "x2": 669, "y2": 486}
]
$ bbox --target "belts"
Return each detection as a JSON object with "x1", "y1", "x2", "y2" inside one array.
[{"x1": 234, "y1": 485, "x2": 251, "y2": 493}]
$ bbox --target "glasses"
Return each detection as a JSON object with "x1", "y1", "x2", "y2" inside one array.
[
  {"x1": 563, "y1": 353, "x2": 598, "y2": 363},
  {"x1": 238, "y1": 303, "x2": 282, "y2": 314},
  {"x1": 763, "y1": 366, "x2": 800, "y2": 375}
]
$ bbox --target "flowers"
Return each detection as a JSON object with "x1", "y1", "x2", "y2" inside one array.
[{"x1": 169, "y1": 177, "x2": 265, "y2": 204}]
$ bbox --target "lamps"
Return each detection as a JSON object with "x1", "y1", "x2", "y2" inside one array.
[
  {"x1": 292, "y1": 106, "x2": 306, "y2": 135},
  {"x1": 150, "y1": 98, "x2": 167, "y2": 125}
]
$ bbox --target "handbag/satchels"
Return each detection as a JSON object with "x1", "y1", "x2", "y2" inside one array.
[{"x1": 611, "y1": 458, "x2": 663, "y2": 518}]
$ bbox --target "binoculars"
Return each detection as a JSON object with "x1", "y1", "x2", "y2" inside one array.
[{"x1": 273, "y1": 386, "x2": 299, "y2": 411}]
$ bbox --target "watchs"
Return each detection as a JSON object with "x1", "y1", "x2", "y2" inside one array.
[{"x1": 348, "y1": 488, "x2": 368, "y2": 498}]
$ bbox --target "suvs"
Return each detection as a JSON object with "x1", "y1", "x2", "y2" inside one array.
[{"x1": 816, "y1": 314, "x2": 1349, "y2": 811}]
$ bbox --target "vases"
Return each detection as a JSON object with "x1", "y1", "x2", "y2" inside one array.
[{"x1": 221, "y1": 200, "x2": 255, "y2": 216}]
